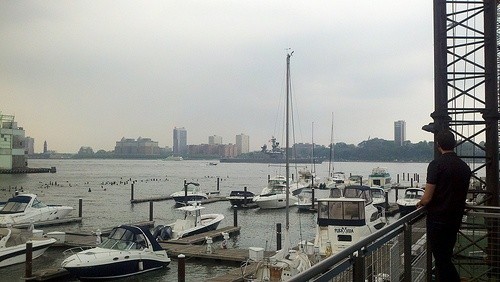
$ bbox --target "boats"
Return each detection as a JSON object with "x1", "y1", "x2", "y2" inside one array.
[
  {"x1": 151, "y1": 202, "x2": 224, "y2": 242},
  {"x1": 226, "y1": 191, "x2": 256, "y2": 206},
  {"x1": 170, "y1": 187, "x2": 208, "y2": 203},
  {"x1": 257, "y1": 198, "x2": 388, "y2": 282},
  {"x1": 253, "y1": 167, "x2": 321, "y2": 209},
  {"x1": 294, "y1": 187, "x2": 341, "y2": 210},
  {"x1": 397, "y1": 188, "x2": 425, "y2": 210},
  {"x1": 61, "y1": 224, "x2": 171, "y2": 278},
  {"x1": 0, "y1": 228, "x2": 57, "y2": 267},
  {"x1": 0, "y1": 194, "x2": 73, "y2": 228},
  {"x1": 326, "y1": 167, "x2": 401, "y2": 223}
]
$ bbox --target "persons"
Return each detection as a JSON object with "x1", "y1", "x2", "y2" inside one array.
[{"x1": 416, "y1": 130, "x2": 471, "y2": 282}]
